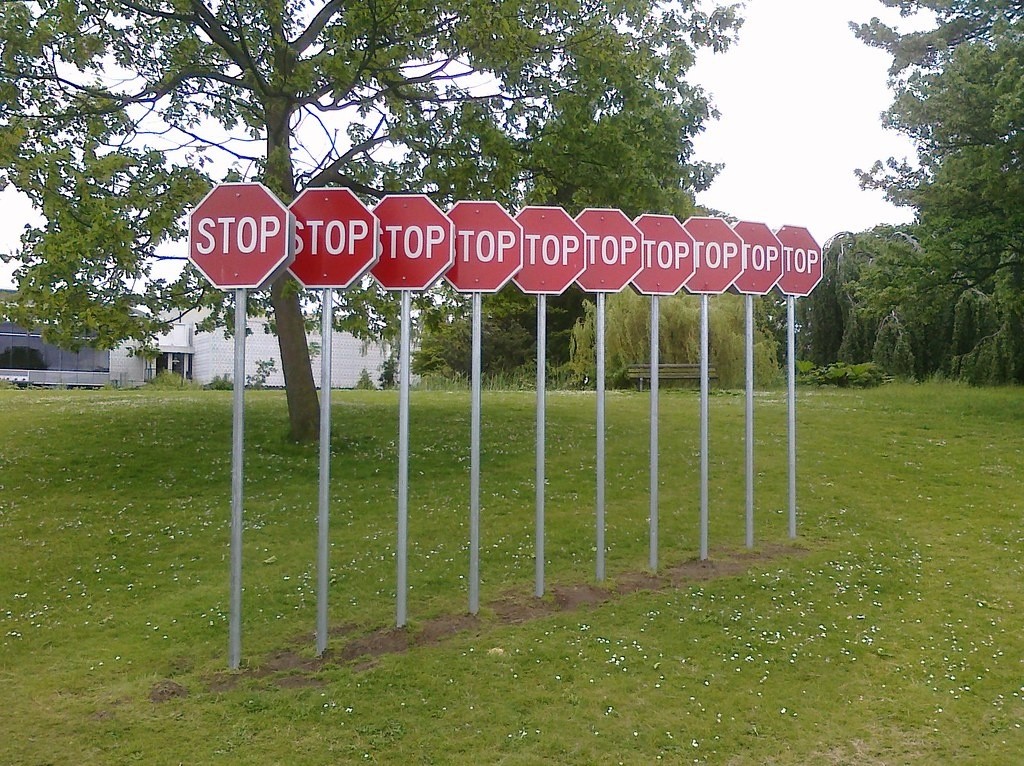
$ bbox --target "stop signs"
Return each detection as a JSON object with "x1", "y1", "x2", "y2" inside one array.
[
  {"x1": 572, "y1": 208, "x2": 645, "y2": 293},
  {"x1": 443, "y1": 199, "x2": 523, "y2": 294},
  {"x1": 187, "y1": 183, "x2": 296, "y2": 292},
  {"x1": 679, "y1": 216, "x2": 744, "y2": 296},
  {"x1": 368, "y1": 194, "x2": 455, "y2": 292},
  {"x1": 726, "y1": 219, "x2": 784, "y2": 296},
  {"x1": 625, "y1": 214, "x2": 696, "y2": 295},
  {"x1": 772, "y1": 224, "x2": 824, "y2": 298},
  {"x1": 282, "y1": 187, "x2": 380, "y2": 290},
  {"x1": 510, "y1": 206, "x2": 586, "y2": 296}
]
